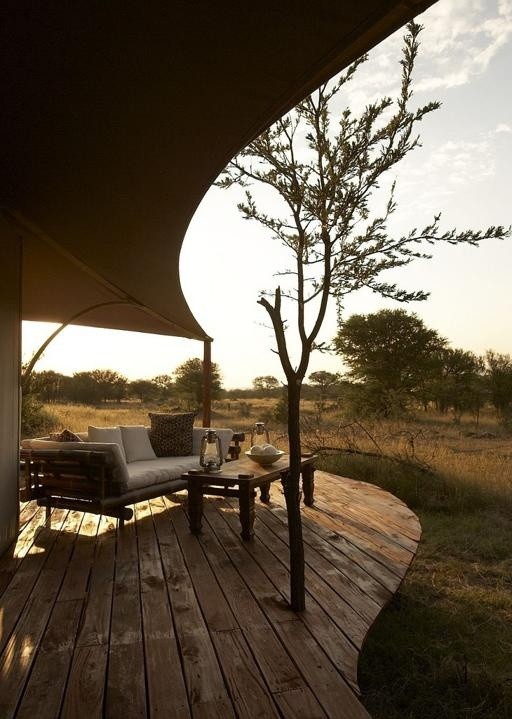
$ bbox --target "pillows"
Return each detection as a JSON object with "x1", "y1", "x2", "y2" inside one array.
[
  {"x1": 119, "y1": 425, "x2": 157, "y2": 463},
  {"x1": 148, "y1": 412, "x2": 195, "y2": 457},
  {"x1": 88, "y1": 425, "x2": 127, "y2": 464},
  {"x1": 49, "y1": 429, "x2": 83, "y2": 442}
]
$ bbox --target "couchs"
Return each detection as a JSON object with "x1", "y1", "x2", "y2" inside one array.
[{"x1": 20, "y1": 428, "x2": 245, "y2": 529}]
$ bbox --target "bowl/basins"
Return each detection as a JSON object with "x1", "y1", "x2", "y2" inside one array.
[{"x1": 245, "y1": 451, "x2": 284, "y2": 466}]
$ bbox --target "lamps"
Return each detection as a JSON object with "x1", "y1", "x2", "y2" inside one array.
[
  {"x1": 251, "y1": 422, "x2": 269, "y2": 448},
  {"x1": 199, "y1": 429, "x2": 223, "y2": 474}
]
